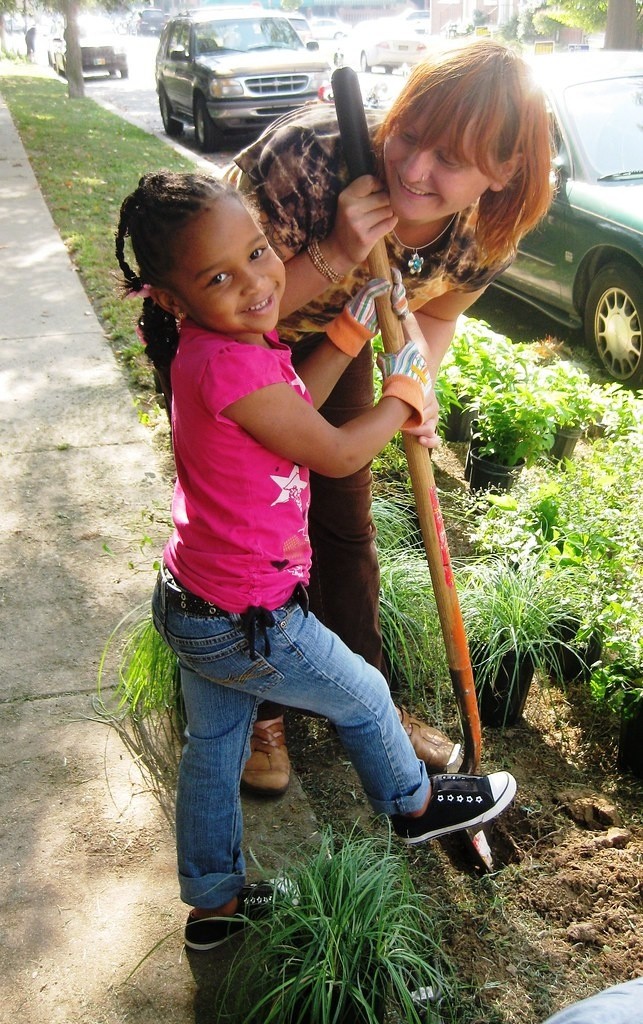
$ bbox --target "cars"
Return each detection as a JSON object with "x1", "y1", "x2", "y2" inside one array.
[
  {"x1": 476, "y1": 47, "x2": 643, "y2": 385},
  {"x1": 38, "y1": 6, "x2": 171, "y2": 79},
  {"x1": 287, "y1": 12, "x2": 507, "y2": 81}
]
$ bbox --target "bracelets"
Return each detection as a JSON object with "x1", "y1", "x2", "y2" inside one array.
[{"x1": 307, "y1": 242, "x2": 345, "y2": 284}]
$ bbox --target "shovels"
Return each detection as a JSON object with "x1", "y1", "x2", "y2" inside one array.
[{"x1": 329, "y1": 67, "x2": 502, "y2": 875}]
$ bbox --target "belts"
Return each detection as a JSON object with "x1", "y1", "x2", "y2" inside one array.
[{"x1": 156, "y1": 556, "x2": 309, "y2": 619}]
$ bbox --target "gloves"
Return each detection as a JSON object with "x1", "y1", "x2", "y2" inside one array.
[
  {"x1": 376, "y1": 339, "x2": 432, "y2": 430},
  {"x1": 324, "y1": 266, "x2": 409, "y2": 357}
]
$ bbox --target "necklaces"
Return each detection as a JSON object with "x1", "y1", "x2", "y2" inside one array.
[{"x1": 392, "y1": 213, "x2": 457, "y2": 274}]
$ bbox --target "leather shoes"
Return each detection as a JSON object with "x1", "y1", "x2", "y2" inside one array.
[
  {"x1": 395, "y1": 700, "x2": 455, "y2": 772},
  {"x1": 240, "y1": 722, "x2": 290, "y2": 799}
]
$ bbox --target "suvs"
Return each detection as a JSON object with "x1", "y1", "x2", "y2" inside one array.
[{"x1": 151, "y1": 7, "x2": 330, "y2": 156}]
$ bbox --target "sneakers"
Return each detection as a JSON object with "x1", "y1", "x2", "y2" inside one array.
[
  {"x1": 390, "y1": 771, "x2": 517, "y2": 843},
  {"x1": 184, "y1": 877, "x2": 300, "y2": 950}
]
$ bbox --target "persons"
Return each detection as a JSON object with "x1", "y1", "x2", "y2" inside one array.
[
  {"x1": 25, "y1": 27, "x2": 36, "y2": 55},
  {"x1": 156, "y1": 42, "x2": 556, "y2": 797},
  {"x1": 116, "y1": 170, "x2": 516, "y2": 951}
]
